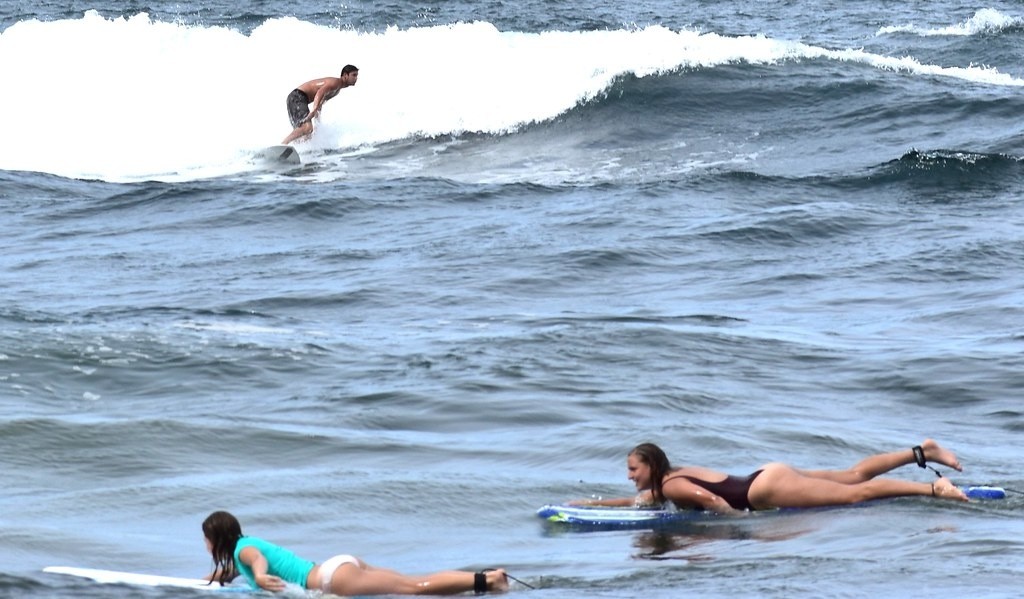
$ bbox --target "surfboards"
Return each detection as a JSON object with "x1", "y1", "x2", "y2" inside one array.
[
  {"x1": 537, "y1": 502, "x2": 835, "y2": 527},
  {"x1": 258, "y1": 144, "x2": 304, "y2": 167},
  {"x1": 40, "y1": 563, "x2": 343, "y2": 599}
]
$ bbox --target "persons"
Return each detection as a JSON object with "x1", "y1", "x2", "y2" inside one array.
[
  {"x1": 202, "y1": 510, "x2": 509, "y2": 596},
  {"x1": 281, "y1": 65, "x2": 359, "y2": 144},
  {"x1": 568, "y1": 438, "x2": 970, "y2": 515}
]
